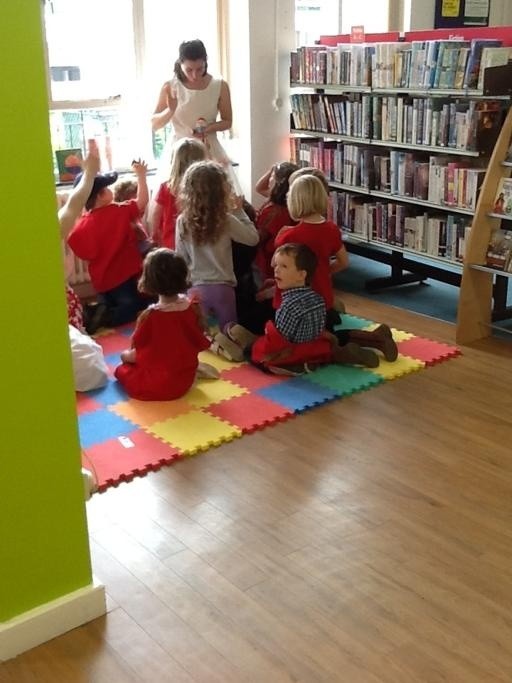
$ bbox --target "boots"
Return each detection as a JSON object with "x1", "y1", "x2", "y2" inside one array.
[{"x1": 343, "y1": 323, "x2": 398, "y2": 362}]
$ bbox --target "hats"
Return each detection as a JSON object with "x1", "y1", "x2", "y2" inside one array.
[{"x1": 73, "y1": 171, "x2": 117, "y2": 191}]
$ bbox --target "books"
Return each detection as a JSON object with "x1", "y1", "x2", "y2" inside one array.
[{"x1": 286, "y1": 34, "x2": 512, "y2": 274}]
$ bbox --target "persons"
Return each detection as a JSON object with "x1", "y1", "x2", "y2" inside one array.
[
  {"x1": 175, "y1": 159, "x2": 261, "y2": 361},
  {"x1": 273, "y1": 173, "x2": 398, "y2": 367},
  {"x1": 150, "y1": 37, "x2": 237, "y2": 198},
  {"x1": 54, "y1": 136, "x2": 347, "y2": 334},
  {"x1": 251, "y1": 241, "x2": 336, "y2": 377},
  {"x1": 112, "y1": 246, "x2": 223, "y2": 402},
  {"x1": 63, "y1": 280, "x2": 111, "y2": 392}
]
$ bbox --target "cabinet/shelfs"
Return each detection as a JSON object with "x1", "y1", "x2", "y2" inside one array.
[{"x1": 289, "y1": 84, "x2": 511, "y2": 347}]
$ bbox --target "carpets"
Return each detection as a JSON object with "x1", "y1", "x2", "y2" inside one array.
[{"x1": 72, "y1": 312, "x2": 464, "y2": 493}]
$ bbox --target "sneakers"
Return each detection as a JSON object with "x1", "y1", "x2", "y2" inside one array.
[
  {"x1": 268, "y1": 364, "x2": 306, "y2": 377},
  {"x1": 85, "y1": 303, "x2": 108, "y2": 336},
  {"x1": 344, "y1": 342, "x2": 379, "y2": 368},
  {"x1": 214, "y1": 333, "x2": 245, "y2": 362},
  {"x1": 196, "y1": 361, "x2": 220, "y2": 379},
  {"x1": 228, "y1": 322, "x2": 258, "y2": 349}
]
